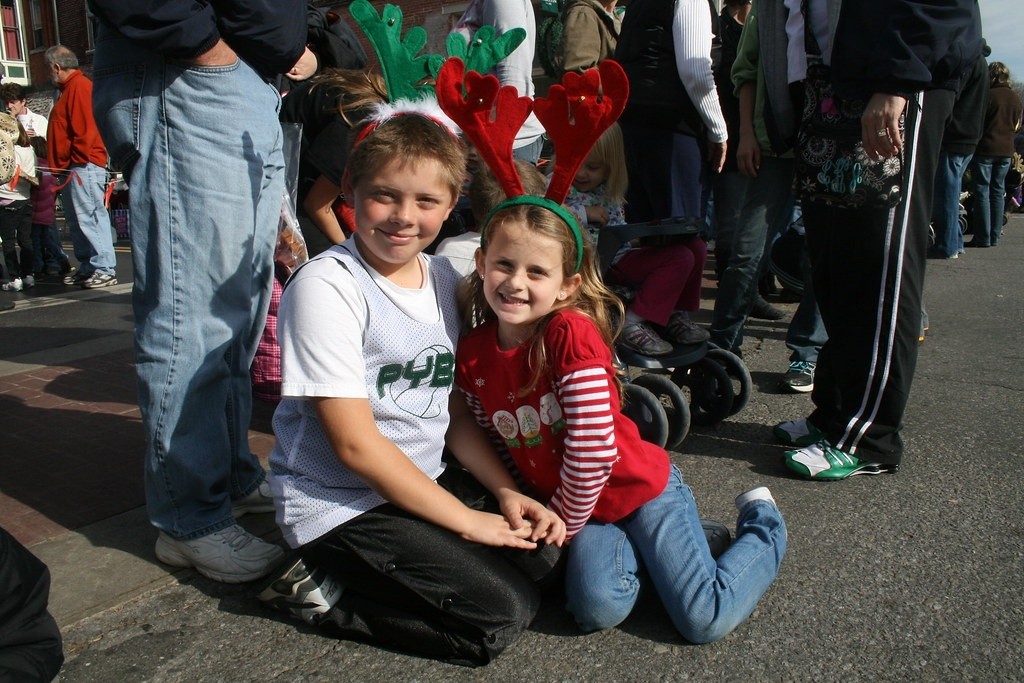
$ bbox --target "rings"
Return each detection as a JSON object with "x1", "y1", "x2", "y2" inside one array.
[
  {"x1": 292, "y1": 67, "x2": 298, "y2": 74},
  {"x1": 875, "y1": 128, "x2": 889, "y2": 138}
]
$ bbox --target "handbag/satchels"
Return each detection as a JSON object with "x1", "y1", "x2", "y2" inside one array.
[{"x1": 794, "y1": 64, "x2": 906, "y2": 210}]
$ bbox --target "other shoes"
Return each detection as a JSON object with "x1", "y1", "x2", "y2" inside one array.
[
  {"x1": 253, "y1": 380, "x2": 282, "y2": 401},
  {"x1": 35, "y1": 271, "x2": 45, "y2": 282},
  {"x1": 990, "y1": 238, "x2": 998, "y2": 245},
  {"x1": 927, "y1": 245, "x2": 964, "y2": 259},
  {"x1": 48, "y1": 268, "x2": 59, "y2": 276},
  {"x1": 963, "y1": 235, "x2": 990, "y2": 247},
  {"x1": 749, "y1": 303, "x2": 786, "y2": 319}
]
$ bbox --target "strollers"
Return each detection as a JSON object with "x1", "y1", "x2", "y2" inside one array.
[{"x1": 579, "y1": 216, "x2": 754, "y2": 448}]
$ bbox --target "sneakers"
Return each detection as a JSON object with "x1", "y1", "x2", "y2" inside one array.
[
  {"x1": 155, "y1": 522, "x2": 285, "y2": 583},
  {"x1": 784, "y1": 437, "x2": 899, "y2": 480},
  {"x1": 1, "y1": 277, "x2": 24, "y2": 292},
  {"x1": 81, "y1": 267, "x2": 118, "y2": 288},
  {"x1": 665, "y1": 311, "x2": 710, "y2": 344},
  {"x1": 255, "y1": 556, "x2": 346, "y2": 626},
  {"x1": 612, "y1": 319, "x2": 674, "y2": 356},
  {"x1": 735, "y1": 486, "x2": 776, "y2": 512},
  {"x1": 773, "y1": 417, "x2": 825, "y2": 447},
  {"x1": 23, "y1": 275, "x2": 35, "y2": 289},
  {"x1": 229, "y1": 470, "x2": 277, "y2": 518},
  {"x1": 780, "y1": 360, "x2": 817, "y2": 392},
  {"x1": 62, "y1": 264, "x2": 95, "y2": 285},
  {"x1": 700, "y1": 519, "x2": 731, "y2": 558}
]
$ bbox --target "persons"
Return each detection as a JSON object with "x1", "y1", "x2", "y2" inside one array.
[
  {"x1": 89, "y1": 1, "x2": 331, "y2": 587},
  {"x1": 436, "y1": 57, "x2": 789, "y2": 645},
  {"x1": 42, "y1": 43, "x2": 118, "y2": 291},
  {"x1": 754, "y1": 1, "x2": 985, "y2": 483},
  {"x1": 253, "y1": 0, "x2": 568, "y2": 668},
  {"x1": 0, "y1": 0, "x2": 1024, "y2": 484}
]
